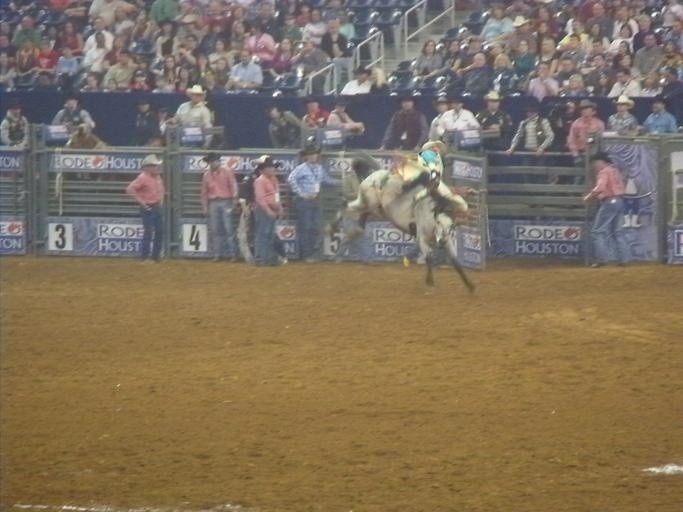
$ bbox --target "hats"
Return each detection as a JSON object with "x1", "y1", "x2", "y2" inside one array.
[
  {"x1": 142, "y1": 156, "x2": 162, "y2": 166},
  {"x1": 589, "y1": 151, "x2": 609, "y2": 163},
  {"x1": 418, "y1": 140, "x2": 442, "y2": 153},
  {"x1": 304, "y1": 95, "x2": 318, "y2": 104},
  {"x1": 63, "y1": 92, "x2": 81, "y2": 102},
  {"x1": 480, "y1": 91, "x2": 503, "y2": 102},
  {"x1": 434, "y1": 97, "x2": 447, "y2": 106},
  {"x1": 651, "y1": 94, "x2": 670, "y2": 109},
  {"x1": 524, "y1": 101, "x2": 539, "y2": 112},
  {"x1": 139, "y1": 99, "x2": 151, "y2": 105},
  {"x1": 300, "y1": 145, "x2": 321, "y2": 156},
  {"x1": 576, "y1": 100, "x2": 596, "y2": 110},
  {"x1": 260, "y1": 157, "x2": 279, "y2": 169},
  {"x1": 399, "y1": 94, "x2": 416, "y2": 102},
  {"x1": 451, "y1": 95, "x2": 467, "y2": 102},
  {"x1": 353, "y1": 67, "x2": 371, "y2": 76},
  {"x1": 332, "y1": 95, "x2": 350, "y2": 106},
  {"x1": 612, "y1": 97, "x2": 635, "y2": 110},
  {"x1": 7, "y1": 98, "x2": 22, "y2": 109},
  {"x1": 204, "y1": 154, "x2": 219, "y2": 163},
  {"x1": 185, "y1": 85, "x2": 206, "y2": 98},
  {"x1": 263, "y1": 101, "x2": 277, "y2": 115},
  {"x1": 511, "y1": 14, "x2": 527, "y2": 28}
]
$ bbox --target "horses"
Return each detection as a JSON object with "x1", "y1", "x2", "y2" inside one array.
[
  {"x1": 325, "y1": 151, "x2": 475, "y2": 293},
  {"x1": 49, "y1": 123, "x2": 139, "y2": 182}
]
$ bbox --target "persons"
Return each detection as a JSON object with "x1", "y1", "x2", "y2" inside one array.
[
  {"x1": 124, "y1": 154, "x2": 165, "y2": 266},
  {"x1": 580, "y1": 152, "x2": 626, "y2": 269},
  {"x1": 238, "y1": 153, "x2": 287, "y2": 266},
  {"x1": 401, "y1": 179, "x2": 480, "y2": 268},
  {"x1": 285, "y1": 144, "x2": 344, "y2": 265},
  {"x1": 200, "y1": 152, "x2": 240, "y2": 264},
  {"x1": 397, "y1": 140, "x2": 444, "y2": 208},
  {"x1": 0, "y1": 1, "x2": 683, "y2": 168}
]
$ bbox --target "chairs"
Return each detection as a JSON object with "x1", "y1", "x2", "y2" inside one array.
[{"x1": 340, "y1": 0, "x2": 486, "y2": 83}]
